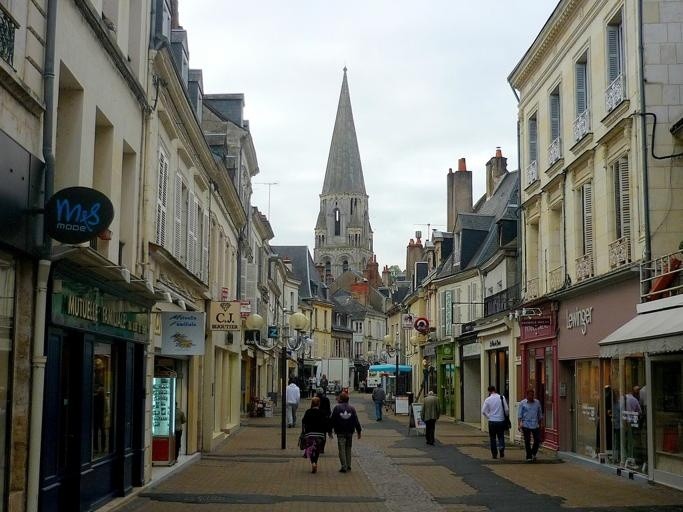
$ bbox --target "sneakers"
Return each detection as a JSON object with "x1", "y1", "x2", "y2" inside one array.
[
  {"x1": 339, "y1": 465, "x2": 351, "y2": 473},
  {"x1": 288, "y1": 425, "x2": 295, "y2": 428},
  {"x1": 493, "y1": 447, "x2": 504, "y2": 459},
  {"x1": 312, "y1": 464, "x2": 317, "y2": 473},
  {"x1": 526, "y1": 455, "x2": 537, "y2": 462}
]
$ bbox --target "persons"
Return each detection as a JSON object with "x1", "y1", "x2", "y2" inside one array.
[
  {"x1": 299, "y1": 396, "x2": 333, "y2": 475},
  {"x1": 284, "y1": 379, "x2": 301, "y2": 429},
  {"x1": 358, "y1": 380, "x2": 366, "y2": 391},
  {"x1": 420, "y1": 390, "x2": 439, "y2": 445},
  {"x1": 618, "y1": 391, "x2": 642, "y2": 428},
  {"x1": 481, "y1": 384, "x2": 510, "y2": 459},
  {"x1": 308, "y1": 373, "x2": 317, "y2": 398},
  {"x1": 517, "y1": 390, "x2": 544, "y2": 462},
  {"x1": 176, "y1": 401, "x2": 185, "y2": 461},
  {"x1": 371, "y1": 382, "x2": 386, "y2": 422},
  {"x1": 319, "y1": 374, "x2": 328, "y2": 397},
  {"x1": 326, "y1": 391, "x2": 362, "y2": 472}
]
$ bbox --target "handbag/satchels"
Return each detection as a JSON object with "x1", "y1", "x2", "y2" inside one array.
[
  {"x1": 297, "y1": 424, "x2": 305, "y2": 450},
  {"x1": 504, "y1": 417, "x2": 512, "y2": 429}
]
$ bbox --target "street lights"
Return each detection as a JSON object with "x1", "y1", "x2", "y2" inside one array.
[
  {"x1": 383, "y1": 332, "x2": 418, "y2": 393},
  {"x1": 245, "y1": 307, "x2": 311, "y2": 449},
  {"x1": 359, "y1": 351, "x2": 376, "y2": 367}
]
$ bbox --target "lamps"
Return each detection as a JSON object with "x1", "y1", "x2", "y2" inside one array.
[
  {"x1": 509, "y1": 307, "x2": 542, "y2": 320},
  {"x1": 80, "y1": 264, "x2": 187, "y2": 311}
]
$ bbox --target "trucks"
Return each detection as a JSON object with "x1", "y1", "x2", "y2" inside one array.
[{"x1": 321, "y1": 357, "x2": 350, "y2": 394}]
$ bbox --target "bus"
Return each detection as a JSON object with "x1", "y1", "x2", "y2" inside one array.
[{"x1": 366, "y1": 363, "x2": 412, "y2": 394}]
